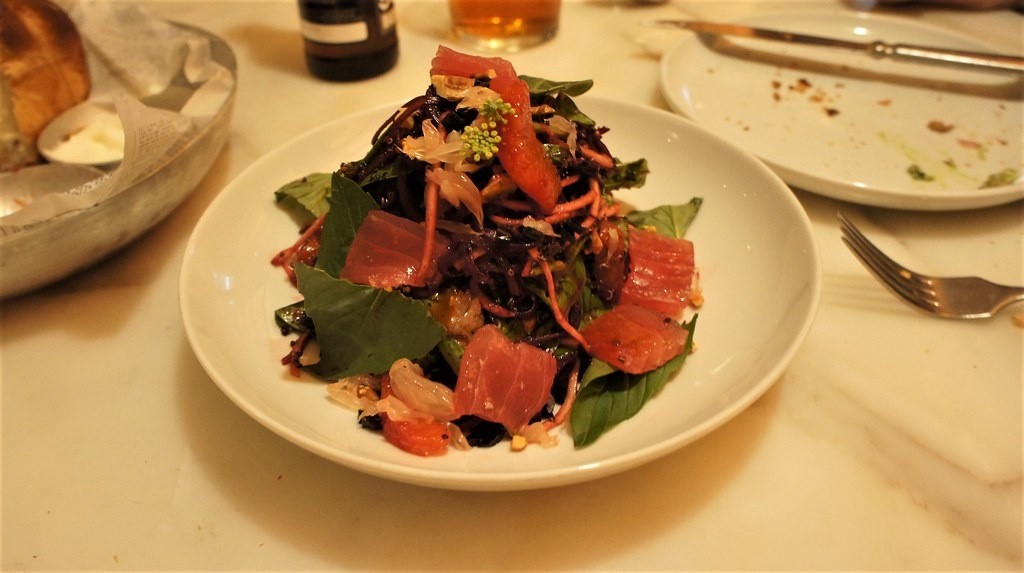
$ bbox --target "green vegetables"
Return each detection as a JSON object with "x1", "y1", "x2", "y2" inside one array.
[{"x1": 276, "y1": 75, "x2": 708, "y2": 451}]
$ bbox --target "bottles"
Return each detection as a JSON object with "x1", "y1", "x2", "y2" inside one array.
[{"x1": 297, "y1": 0, "x2": 400, "y2": 83}]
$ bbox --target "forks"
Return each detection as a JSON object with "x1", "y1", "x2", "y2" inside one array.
[{"x1": 836, "y1": 211, "x2": 1024, "y2": 320}]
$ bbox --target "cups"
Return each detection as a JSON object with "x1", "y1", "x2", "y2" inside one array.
[{"x1": 446, "y1": 0, "x2": 561, "y2": 55}]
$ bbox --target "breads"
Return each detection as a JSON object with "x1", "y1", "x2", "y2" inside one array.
[{"x1": 0, "y1": 0, "x2": 91, "y2": 173}]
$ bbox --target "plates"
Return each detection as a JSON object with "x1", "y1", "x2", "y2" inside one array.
[
  {"x1": 659, "y1": 7, "x2": 1024, "y2": 211},
  {"x1": 179, "y1": 92, "x2": 821, "y2": 491}
]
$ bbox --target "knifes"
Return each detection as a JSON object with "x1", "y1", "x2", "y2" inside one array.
[{"x1": 652, "y1": 17, "x2": 1024, "y2": 77}]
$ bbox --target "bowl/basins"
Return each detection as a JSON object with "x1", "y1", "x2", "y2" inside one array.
[{"x1": 0, "y1": 18, "x2": 239, "y2": 303}]
